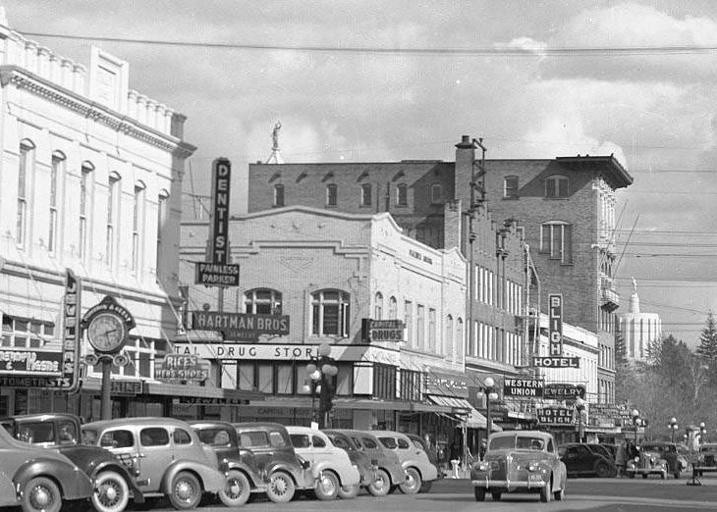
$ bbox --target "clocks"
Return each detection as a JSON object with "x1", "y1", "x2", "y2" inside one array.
[{"x1": 87, "y1": 312, "x2": 127, "y2": 352}]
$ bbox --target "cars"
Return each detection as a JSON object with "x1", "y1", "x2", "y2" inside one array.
[
  {"x1": 185, "y1": 418, "x2": 261, "y2": 507},
  {"x1": 320, "y1": 429, "x2": 443, "y2": 498},
  {"x1": 231, "y1": 419, "x2": 317, "y2": 504},
  {"x1": 557, "y1": 442, "x2": 717, "y2": 480},
  {"x1": 79, "y1": 415, "x2": 228, "y2": 511},
  {"x1": 285, "y1": 424, "x2": 361, "y2": 503},
  {"x1": 469, "y1": 429, "x2": 569, "y2": 503},
  {"x1": 0, "y1": 411, "x2": 146, "y2": 511}
]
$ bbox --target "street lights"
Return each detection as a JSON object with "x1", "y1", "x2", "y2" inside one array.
[
  {"x1": 475, "y1": 376, "x2": 500, "y2": 448},
  {"x1": 667, "y1": 417, "x2": 679, "y2": 442},
  {"x1": 303, "y1": 341, "x2": 339, "y2": 430},
  {"x1": 699, "y1": 422, "x2": 707, "y2": 444},
  {"x1": 627, "y1": 408, "x2": 643, "y2": 447}
]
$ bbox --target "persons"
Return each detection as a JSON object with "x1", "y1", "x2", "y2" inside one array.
[
  {"x1": 450, "y1": 442, "x2": 461, "y2": 479},
  {"x1": 691, "y1": 432, "x2": 703, "y2": 479}
]
